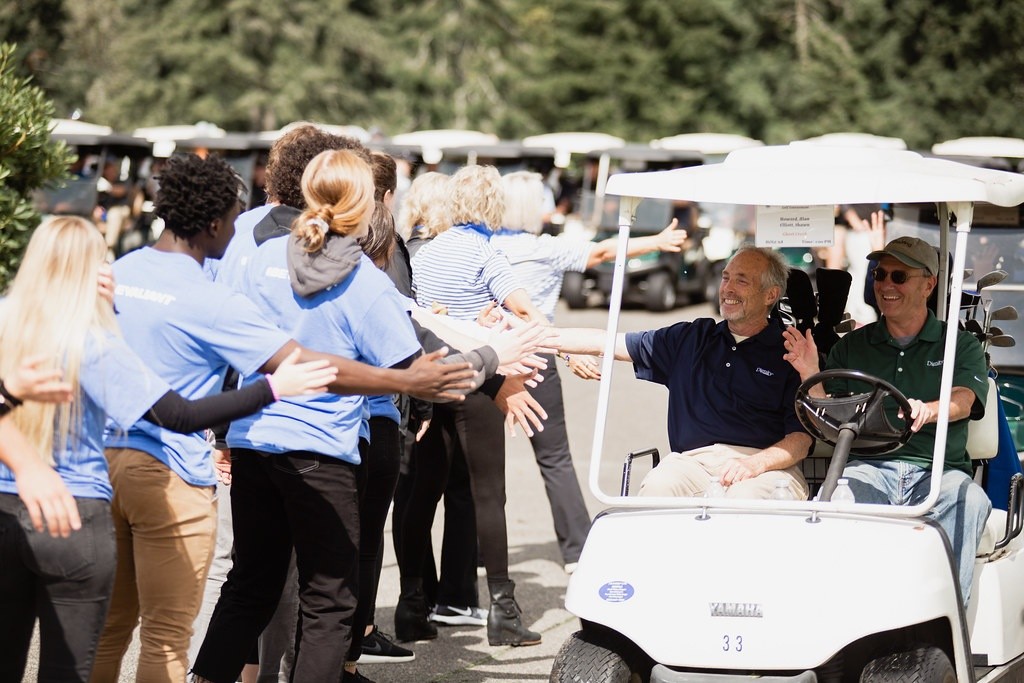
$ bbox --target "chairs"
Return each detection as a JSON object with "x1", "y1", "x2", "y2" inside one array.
[{"x1": 785, "y1": 366, "x2": 1024, "y2": 552}]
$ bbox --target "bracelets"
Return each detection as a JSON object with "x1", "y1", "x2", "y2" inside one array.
[
  {"x1": 265, "y1": 373, "x2": 280, "y2": 403},
  {"x1": 566, "y1": 354, "x2": 570, "y2": 366}
]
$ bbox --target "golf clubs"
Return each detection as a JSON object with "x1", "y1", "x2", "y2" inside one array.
[{"x1": 943, "y1": 266, "x2": 1018, "y2": 361}]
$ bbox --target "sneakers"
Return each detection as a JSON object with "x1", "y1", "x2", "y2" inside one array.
[
  {"x1": 429, "y1": 603, "x2": 489, "y2": 624},
  {"x1": 342, "y1": 668, "x2": 375, "y2": 683},
  {"x1": 356, "y1": 625, "x2": 416, "y2": 664}
]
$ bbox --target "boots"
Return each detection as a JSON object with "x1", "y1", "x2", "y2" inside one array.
[
  {"x1": 395, "y1": 578, "x2": 437, "y2": 640},
  {"x1": 488, "y1": 580, "x2": 541, "y2": 646}
]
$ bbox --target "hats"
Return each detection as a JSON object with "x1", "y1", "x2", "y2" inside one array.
[{"x1": 866, "y1": 236, "x2": 939, "y2": 277}]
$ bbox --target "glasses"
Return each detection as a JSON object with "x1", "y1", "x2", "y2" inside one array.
[{"x1": 871, "y1": 263, "x2": 930, "y2": 284}]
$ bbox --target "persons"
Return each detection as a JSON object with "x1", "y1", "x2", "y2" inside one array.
[
  {"x1": 491, "y1": 243, "x2": 817, "y2": 502},
  {"x1": 0, "y1": 127, "x2": 688, "y2": 683},
  {"x1": 782, "y1": 235, "x2": 993, "y2": 614},
  {"x1": 820, "y1": 200, "x2": 888, "y2": 331}
]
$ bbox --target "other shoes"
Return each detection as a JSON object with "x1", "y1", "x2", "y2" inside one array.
[{"x1": 564, "y1": 562, "x2": 579, "y2": 573}]
